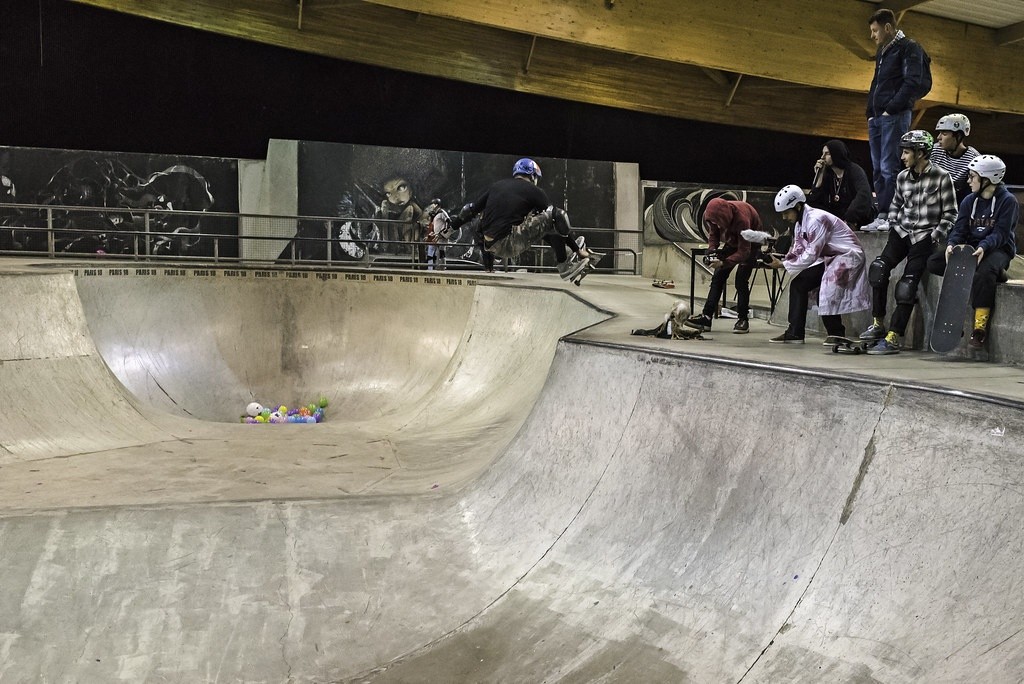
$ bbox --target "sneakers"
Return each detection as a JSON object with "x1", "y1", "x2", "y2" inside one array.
[
  {"x1": 822, "y1": 336, "x2": 845, "y2": 346},
  {"x1": 686, "y1": 314, "x2": 712, "y2": 331},
  {"x1": 558, "y1": 258, "x2": 590, "y2": 281},
  {"x1": 561, "y1": 236, "x2": 584, "y2": 264},
  {"x1": 866, "y1": 338, "x2": 900, "y2": 355},
  {"x1": 733, "y1": 319, "x2": 750, "y2": 334},
  {"x1": 877, "y1": 218, "x2": 890, "y2": 231},
  {"x1": 768, "y1": 332, "x2": 804, "y2": 344},
  {"x1": 970, "y1": 329, "x2": 987, "y2": 348},
  {"x1": 859, "y1": 218, "x2": 885, "y2": 231},
  {"x1": 859, "y1": 325, "x2": 886, "y2": 340}
]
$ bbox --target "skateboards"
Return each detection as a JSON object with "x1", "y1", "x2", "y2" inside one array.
[
  {"x1": 826, "y1": 334, "x2": 885, "y2": 354},
  {"x1": 569, "y1": 242, "x2": 597, "y2": 287},
  {"x1": 930, "y1": 244, "x2": 978, "y2": 354}
]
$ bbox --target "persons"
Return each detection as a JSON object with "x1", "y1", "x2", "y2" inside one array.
[
  {"x1": 424, "y1": 157, "x2": 591, "y2": 281},
  {"x1": 764, "y1": 185, "x2": 866, "y2": 346},
  {"x1": 480, "y1": 245, "x2": 494, "y2": 273},
  {"x1": 930, "y1": 113, "x2": 980, "y2": 195},
  {"x1": 685, "y1": 198, "x2": 762, "y2": 334},
  {"x1": 424, "y1": 211, "x2": 446, "y2": 271},
  {"x1": 859, "y1": 9, "x2": 923, "y2": 230},
  {"x1": 809, "y1": 140, "x2": 877, "y2": 232},
  {"x1": 859, "y1": 129, "x2": 958, "y2": 355},
  {"x1": 928, "y1": 154, "x2": 1021, "y2": 347}
]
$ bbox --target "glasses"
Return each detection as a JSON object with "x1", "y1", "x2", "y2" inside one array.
[{"x1": 967, "y1": 174, "x2": 977, "y2": 179}]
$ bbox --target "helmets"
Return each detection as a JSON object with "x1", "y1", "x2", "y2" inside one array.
[
  {"x1": 773, "y1": 185, "x2": 807, "y2": 212},
  {"x1": 967, "y1": 154, "x2": 1006, "y2": 185},
  {"x1": 512, "y1": 157, "x2": 543, "y2": 180},
  {"x1": 898, "y1": 129, "x2": 934, "y2": 153},
  {"x1": 935, "y1": 113, "x2": 971, "y2": 137}
]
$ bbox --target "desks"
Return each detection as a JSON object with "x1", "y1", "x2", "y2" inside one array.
[{"x1": 690, "y1": 248, "x2": 787, "y2": 316}]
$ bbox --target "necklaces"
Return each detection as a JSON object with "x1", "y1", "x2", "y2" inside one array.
[{"x1": 833, "y1": 174, "x2": 843, "y2": 201}]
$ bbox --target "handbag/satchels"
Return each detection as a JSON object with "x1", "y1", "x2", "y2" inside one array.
[{"x1": 903, "y1": 37, "x2": 933, "y2": 99}]
